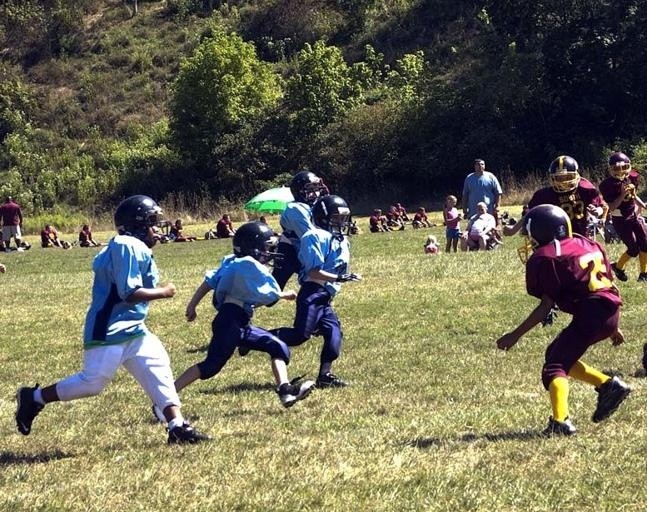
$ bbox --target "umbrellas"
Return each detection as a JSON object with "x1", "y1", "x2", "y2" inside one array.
[{"x1": 244, "y1": 184, "x2": 295, "y2": 227}]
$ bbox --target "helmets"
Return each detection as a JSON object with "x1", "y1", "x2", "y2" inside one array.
[
  {"x1": 517, "y1": 204, "x2": 572, "y2": 265},
  {"x1": 114, "y1": 195, "x2": 168, "y2": 247},
  {"x1": 233, "y1": 221, "x2": 284, "y2": 270},
  {"x1": 313, "y1": 195, "x2": 355, "y2": 242},
  {"x1": 549, "y1": 156, "x2": 581, "y2": 194},
  {"x1": 608, "y1": 152, "x2": 631, "y2": 181},
  {"x1": 290, "y1": 171, "x2": 330, "y2": 207}
]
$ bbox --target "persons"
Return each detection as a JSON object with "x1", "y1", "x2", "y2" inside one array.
[
  {"x1": 253, "y1": 170, "x2": 361, "y2": 313},
  {"x1": 151, "y1": 221, "x2": 317, "y2": 422},
  {"x1": 260, "y1": 215, "x2": 268, "y2": 224},
  {"x1": 0, "y1": 196, "x2": 24, "y2": 253},
  {"x1": 216, "y1": 214, "x2": 237, "y2": 237},
  {"x1": 169, "y1": 218, "x2": 195, "y2": 242},
  {"x1": 78, "y1": 225, "x2": 102, "y2": 247},
  {"x1": 41, "y1": 224, "x2": 65, "y2": 249},
  {"x1": 238, "y1": 194, "x2": 362, "y2": 387},
  {"x1": 15, "y1": 193, "x2": 213, "y2": 442}
]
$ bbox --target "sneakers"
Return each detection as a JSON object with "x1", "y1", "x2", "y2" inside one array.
[
  {"x1": 594, "y1": 375, "x2": 630, "y2": 423},
  {"x1": 168, "y1": 422, "x2": 216, "y2": 444},
  {"x1": 316, "y1": 373, "x2": 349, "y2": 390},
  {"x1": 537, "y1": 415, "x2": 577, "y2": 439},
  {"x1": 542, "y1": 308, "x2": 555, "y2": 327},
  {"x1": 637, "y1": 272, "x2": 647, "y2": 282},
  {"x1": 610, "y1": 262, "x2": 628, "y2": 281},
  {"x1": 16, "y1": 387, "x2": 44, "y2": 435},
  {"x1": 643, "y1": 343, "x2": 647, "y2": 370},
  {"x1": 278, "y1": 380, "x2": 316, "y2": 408},
  {"x1": 152, "y1": 403, "x2": 165, "y2": 421}
]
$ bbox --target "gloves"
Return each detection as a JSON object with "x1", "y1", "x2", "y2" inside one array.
[{"x1": 336, "y1": 274, "x2": 362, "y2": 282}]
